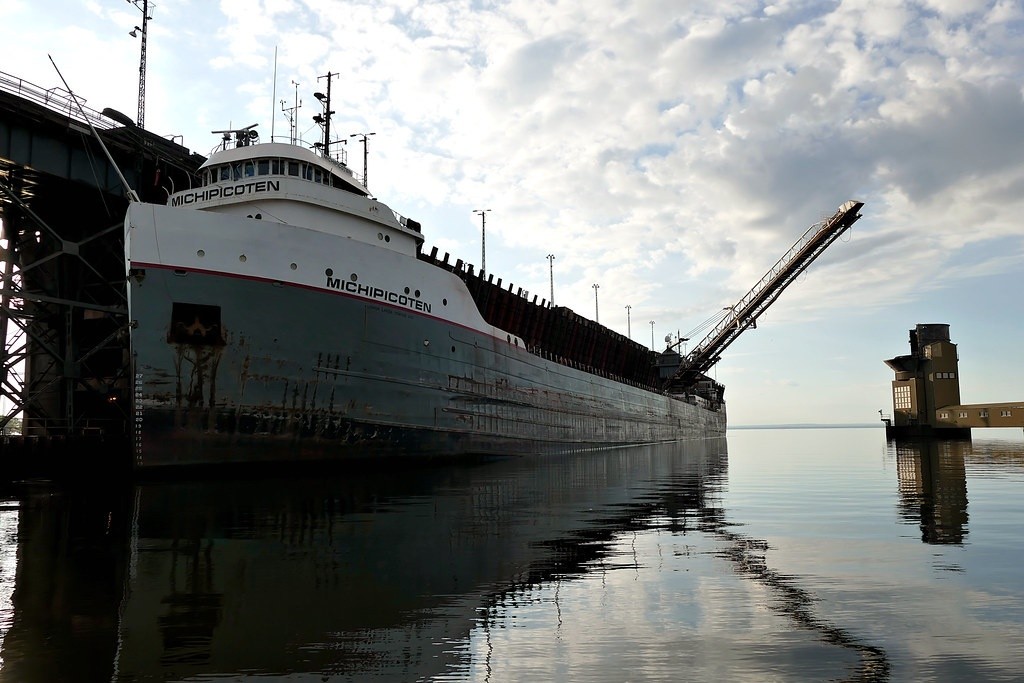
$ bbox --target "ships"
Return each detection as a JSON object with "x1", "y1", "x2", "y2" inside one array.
[{"x1": 118, "y1": 45, "x2": 728, "y2": 472}]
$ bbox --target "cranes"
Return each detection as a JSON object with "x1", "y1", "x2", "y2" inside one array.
[{"x1": 650, "y1": 197, "x2": 867, "y2": 402}]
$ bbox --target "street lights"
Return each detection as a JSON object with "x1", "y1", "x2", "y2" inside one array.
[
  {"x1": 591, "y1": 284, "x2": 600, "y2": 324},
  {"x1": 472, "y1": 209, "x2": 492, "y2": 280},
  {"x1": 350, "y1": 132, "x2": 377, "y2": 196},
  {"x1": 649, "y1": 320, "x2": 656, "y2": 352},
  {"x1": 546, "y1": 254, "x2": 555, "y2": 310},
  {"x1": 625, "y1": 305, "x2": 631, "y2": 341}
]
